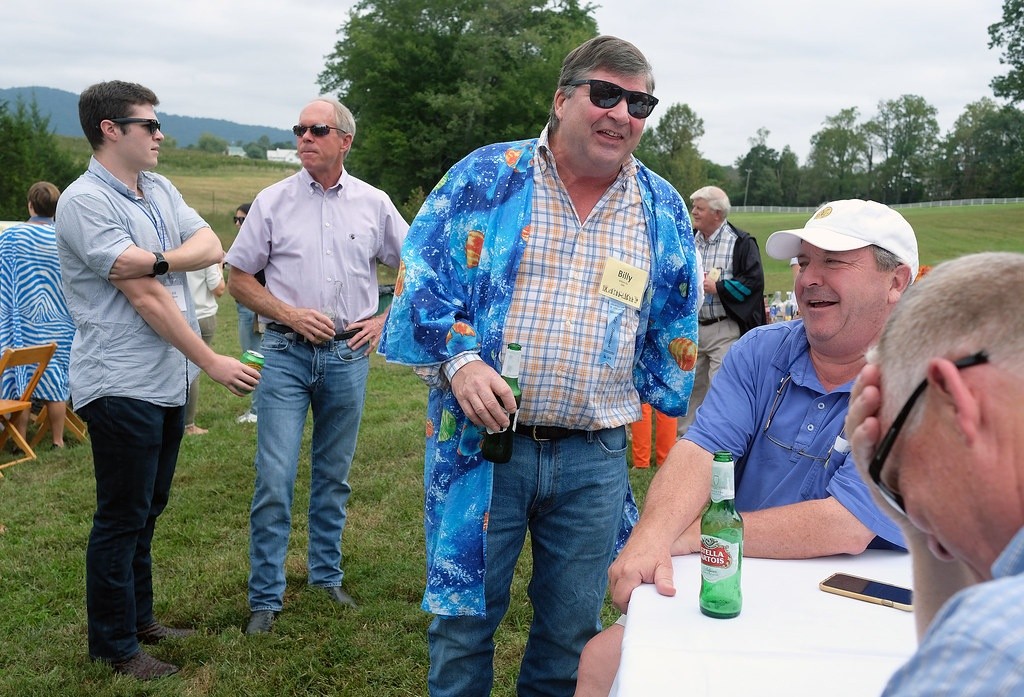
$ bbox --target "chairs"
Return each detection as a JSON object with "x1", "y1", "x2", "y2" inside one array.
[
  {"x1": 0, "y1": 342, "x2": 58, "y2": 478},
  {"x1": 30, "y1": 398, "x2": 89, "y2": 449}
]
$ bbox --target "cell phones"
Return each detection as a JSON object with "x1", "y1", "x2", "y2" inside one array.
[{"x1": 819, "y1": 572, "x2": 914, "y2": 612}]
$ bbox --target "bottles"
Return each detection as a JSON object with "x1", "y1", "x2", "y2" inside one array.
[
  {"x1": 697, "y1": 447, "x2": 745, "y2": 621},
  {"x1": 763, "y1": 289, "x2": 799, "y2": 324},
  {"x1": 479, "y1": 341, "x2": 523, "y2": 466}
]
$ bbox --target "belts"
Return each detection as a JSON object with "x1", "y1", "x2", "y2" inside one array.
[
  {"x1": 265, "y1": 322, "x2": 363, "y2": 344},
  {"x1": 698, "y1": 316, "x2": 727, "y2": 326},
  {"x1": 514, "y1": 423, "x2": 593, "y2": 441}
]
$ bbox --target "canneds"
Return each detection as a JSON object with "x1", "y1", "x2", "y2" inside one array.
[{"x1": 236, "y1": 350, "x2": 264, "y2": 394}]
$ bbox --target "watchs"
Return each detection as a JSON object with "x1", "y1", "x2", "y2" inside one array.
[{"x1": 151, "y1": 252, "x2": 169, "y2": 278}]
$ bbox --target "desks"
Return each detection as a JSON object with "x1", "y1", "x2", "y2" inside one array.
[{"x1": 608, "y1": 549, "x2": 920, "y2": 697}]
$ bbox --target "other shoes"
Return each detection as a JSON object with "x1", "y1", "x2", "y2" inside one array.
[{"x1": 184, "y1": 425, "x2": 208, "y2": 437}]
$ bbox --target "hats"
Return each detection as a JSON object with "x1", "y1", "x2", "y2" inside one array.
[{"x1": 765, "y1": 198, "x2": 918, "y2": 285}]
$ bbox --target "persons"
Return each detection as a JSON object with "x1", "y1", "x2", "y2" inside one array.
[
  {"x1": 377, "y1": 39, "x2": 701, "y2": 697},
  {"x1": 572, "y1": 198, "x2": 921, "y2": 697},
  {"x1": 631, "y1": 403, "x2": 678, "y2": 469},
  {"x1": 232, "y1": 202, "x2": 264, "y2": 422},
  {"x1": 26, "y1": 182, "x2": 60, "y2": 224},
  {"x1": 185, "y1": 265, "x2": 225, "y2": 435},
  {"x1": 846, "y1": 251, "x2": 1024, "y2": 696},
  {"x1": 679, "y1": 185, "x2": 768, "y2": 433},
  {"x1": 52, "y1": 81, "x2": 265, "y2": 682},
  {"x1": 226, "y1": 100, "x2": 413, "y2": 640}
]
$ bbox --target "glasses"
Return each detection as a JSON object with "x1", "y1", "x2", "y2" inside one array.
[
  {"x1": 96, "y1": 117, "x2": 160, "y2": 134},
  {"x1": 764, "y1": 374, "x2": 846, "y2": 460},
  {"x1": 234, "y1": 216, "x2": 245, "y2": 225},
  {"x1": 293, "y1": 124, "x2": 346, "y2": 137},
  {"x1": 564, "y1": 79, "x2": 659, "y2": 119},
  {"x1": 869, "y1": 350, "x2": 988, "y2": 518}
]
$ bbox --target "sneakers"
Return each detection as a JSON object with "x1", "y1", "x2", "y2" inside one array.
[
  {"x1": 325, "y1": 586, "x2": 357, "y2": 609},
  {"x1": 245, "y1": 610, "x2": 278, "y2": 634},
  {"x1": 235, "y1": 410, "x2": 257, "y2": 423},
  {"x1": 136, "y1": 622, "x2": 196, "y2": 645},
  {"x1": 111, "y1": 649, "x2": 179, "y2": 681}
]
